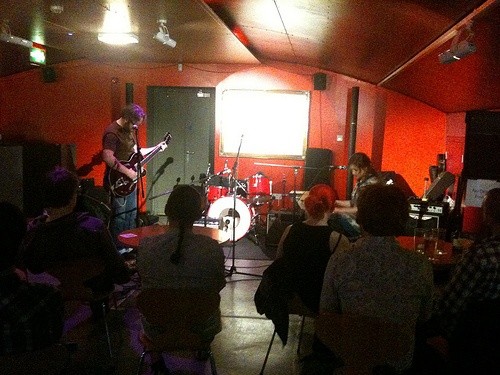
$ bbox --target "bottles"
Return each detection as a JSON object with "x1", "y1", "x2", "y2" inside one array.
[{"x1": 451, "y1": 215, "x2": 463, "y2": 254}]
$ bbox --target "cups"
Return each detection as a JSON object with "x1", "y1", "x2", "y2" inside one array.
[
  {"x1": 423, "y1": 229, "x2": 437, "y2": 260},
  {"x1": 433, "y1": 228, "x2": 447, "y2": 254},
  {"x1": 413, "y1": 227, "x2": 426, "y2": 254}
]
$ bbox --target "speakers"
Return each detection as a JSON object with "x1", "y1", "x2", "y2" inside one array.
[
  {"x1": 268, "y1": 214, "x2": 301, "y2": 246},
  {"x1": 406, "y1": 214, "x2": 439, "y2": 235},
  {"x1": 304, "y1": 149, "x2": 330, "y2": 190},
  {"x1": 314, "y1": 72, "x2": 326, "y2": 90},
  {"x1": 42, "y1": 67, "x2": 55, "y2": 83}
]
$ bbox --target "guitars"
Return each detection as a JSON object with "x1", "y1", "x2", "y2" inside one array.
[{"x1": 104, "y1": 132, "x2": 173, "y2": 197}]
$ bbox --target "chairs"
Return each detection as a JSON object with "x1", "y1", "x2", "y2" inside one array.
[
  {"x1": 257, "y1": 292, "x2": 408, "y2": 375},
  {"x1": 0, "y1": 258, "x2": 223, "y2": 375}
]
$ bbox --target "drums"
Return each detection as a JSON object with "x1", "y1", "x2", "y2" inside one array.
[
  {"x1": 207, "y1": 185, "x2": 227, "y2": 204},
  {"x1": 249, "y1": 175, "x2": 272, "y2": 206},
  {"x1": 205, "y1": 194, "x2": 255, "y2": 242}
]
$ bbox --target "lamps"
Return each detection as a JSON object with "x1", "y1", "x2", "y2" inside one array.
[
  {"x1": 153, "y1": 18, "x2": 177, "y2": 47},
  {"x1": 440, "y1": 22, "x2": 475, "y2": 64}
]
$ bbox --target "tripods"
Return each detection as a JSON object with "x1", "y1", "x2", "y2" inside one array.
[{"x1": 225, "y1": 138, "x2": 263, "y2": 278}]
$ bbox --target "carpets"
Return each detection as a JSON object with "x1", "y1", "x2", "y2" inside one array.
[{"x1": 222, "y1": 237, "x2": 271, "y2": 260}]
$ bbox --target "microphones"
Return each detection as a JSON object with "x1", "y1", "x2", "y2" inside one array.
[
  {"x1": 157, "y1": 157, "x2": 174, "y2": 172},
  {"x1": 133, "y1": 125, "x2": 139, "y2": 142}
]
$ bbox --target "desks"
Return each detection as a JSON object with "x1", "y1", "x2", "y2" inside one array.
[
  {"x1": 394, "y1": 235, "x2": 473, "y2": 266},
  {"x1": 118, "y1": 224, "x2": 233, "y2": 247}
]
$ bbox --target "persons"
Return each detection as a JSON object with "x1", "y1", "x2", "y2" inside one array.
[
  {"x1": 137, "y1": 185, "x2": 227, "y2": 375},
  {"x1": 428, "y1": 188, "x2": 500, "y2": 339},
  {"x1": 334, "y1": 152, "x2": 381, "y2": 213},
  {"x1": 276, "y1": 184, "x2": 350, "y2": 350},
  {"x1": 14, "y1": 164, "x2": 131, "y2": 343},
  {"x1": 312, "y1": 182, "x2": 434, "y2": 375},
  {"x1": 103, "y1": 103, "x2": 146, "y2": 254}
]
